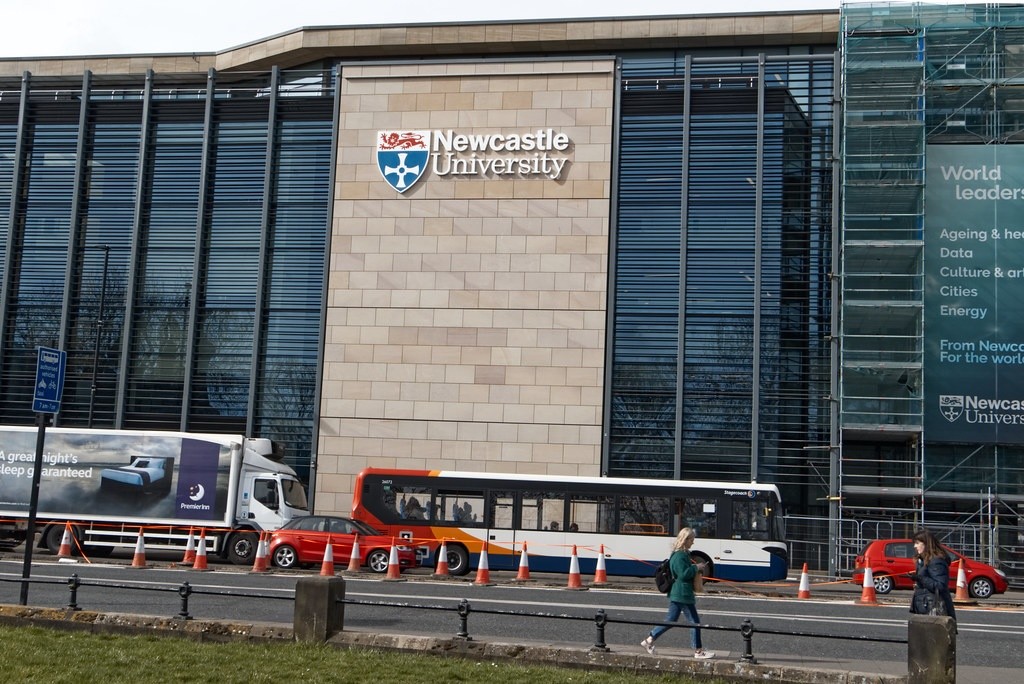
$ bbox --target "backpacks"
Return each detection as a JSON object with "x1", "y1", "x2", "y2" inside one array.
[{"x1": 654, "y1": 551, "x2": 678, "y2": 593}]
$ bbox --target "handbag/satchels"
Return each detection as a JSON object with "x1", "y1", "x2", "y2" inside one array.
[{"x1": 924, "y1": 587, "x2": 948, "y2": 617}]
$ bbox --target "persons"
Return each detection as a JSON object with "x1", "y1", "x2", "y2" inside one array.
[
  {"x1": 457, "y1": 501, "x2": 477, "y2": 521},
  {"x1": 550, "y1": 521, "x2": 559, "y2": 530},
  {"x1": 640, "y1": 527, "x2": 716, "y2": 660},
  {"x1": 907, "y1": 531, "x2": 956, "y2": 620},
  {"x1": 570, "y1": 523, "x2": 578, "y2": 531}
]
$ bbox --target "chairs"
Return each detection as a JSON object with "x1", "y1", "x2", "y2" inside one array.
[
  {"x1": 331, "y1": 523, "x2": 345, "y2": 533},
  {"x1": 400, "y1": 499, "x2": 465, "y2": 523},
  {"x1": 888, "y1": 546, "x2": 896, "y2": 554}
]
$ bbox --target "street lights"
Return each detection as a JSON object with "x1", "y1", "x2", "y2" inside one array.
[
  {"x1": 179, "y1": 282, "x2": 192, "y2": 376},
  {"x1": 86, "y1": 245, "x2": 110, "y2": 428}
]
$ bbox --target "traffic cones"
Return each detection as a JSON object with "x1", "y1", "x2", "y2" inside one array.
[
  {"x1": 510, "y1": 541, "x2": 537, "y2": 582},
  {"x1": 247, "y1": 530, "x2": 274, "y2": 574},
  {"x1": 320, "y1": 533, "x2": 365, "y2": 576},
  {"x1": 793, "y1": 562, "x2": 816, "y2": 601},
  {"x1": 124, "y1": 526, "x2": 153, "y2": 569},
  {"x1": 175, "y1": 526, "x2": 216, "y2": 572},
  {"x1": 383, "y1": 536, "x2": 408, "y2": 582},
  {"x1": 951, "y1": 558, "x2": 977, "y2": 603},
  {"x1": 854, "y1": 557, "x2": 883, "y2": 605},
  {"x1": 562, "y1": 545, "x2": 589, "y2": 591},
  {"x1": 588, "y1": 544, "x2": 613, "y2": 585},
  {"x1": 430, "y1": 537, "x2": 453, "y2": 577},
  {"x1": 51, "y1": 521, "x2": 76, "y2": 558},
  {"x1": 468, "y1": 541, "x2": 498, "y2": 586}
]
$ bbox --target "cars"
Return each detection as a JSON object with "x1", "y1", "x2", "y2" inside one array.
[
  {"x1": 853, "y1": 540, "x2": 1009, "y2": 599},
  {"x1": 268, "y1": 516, "x2": 423, "y2": 574}
]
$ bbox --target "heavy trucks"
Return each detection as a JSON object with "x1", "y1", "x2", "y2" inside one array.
[{"x1": 0, "y1": 425, "x2": 310, "y2": 565}]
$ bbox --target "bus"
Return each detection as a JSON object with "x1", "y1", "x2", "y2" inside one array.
[{"x1": 352, "y1": 466, "x2": 789, "y2": 585}]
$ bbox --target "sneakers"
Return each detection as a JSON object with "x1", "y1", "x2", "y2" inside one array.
[
  {"x1": 694, "y1": 650, "x2": 716, "y2": 659},
  {"x1": 640, "y1": 639, "x2": 658, "y2": 655}
]
